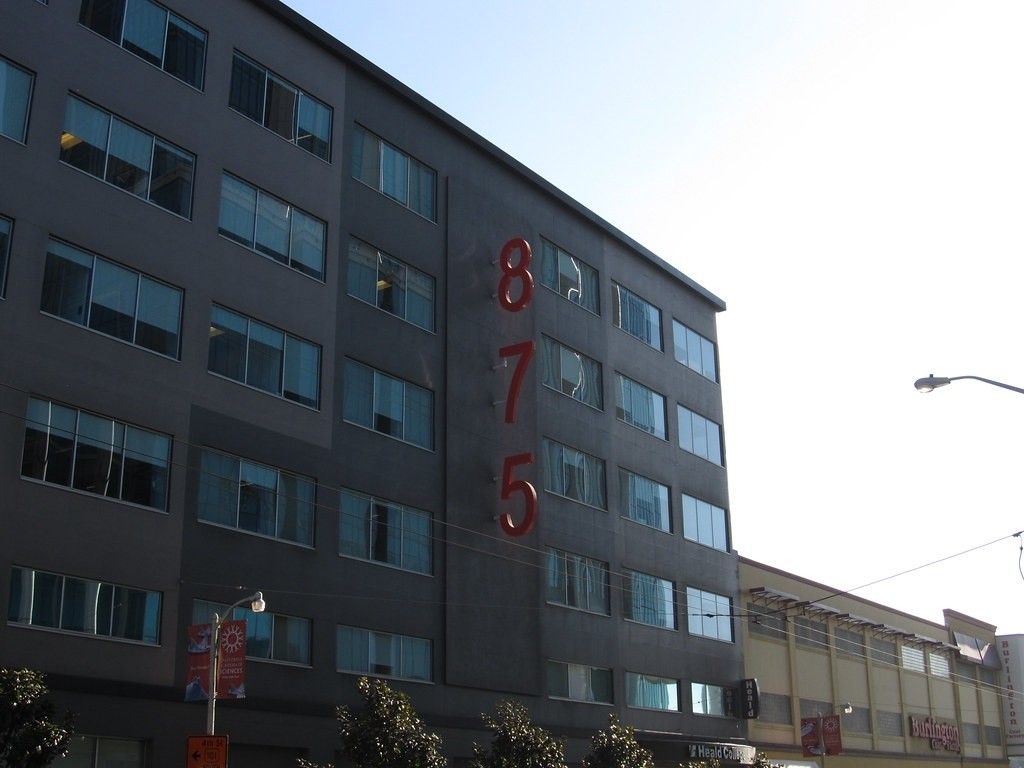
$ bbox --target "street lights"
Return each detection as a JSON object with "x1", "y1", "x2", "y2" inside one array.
[
  {"x1": 817, "y1": 702, "x2": 852, "y2": 768},
  {"x1": 205, "y1": 591, "x2": 265, "y2": 736}
]
